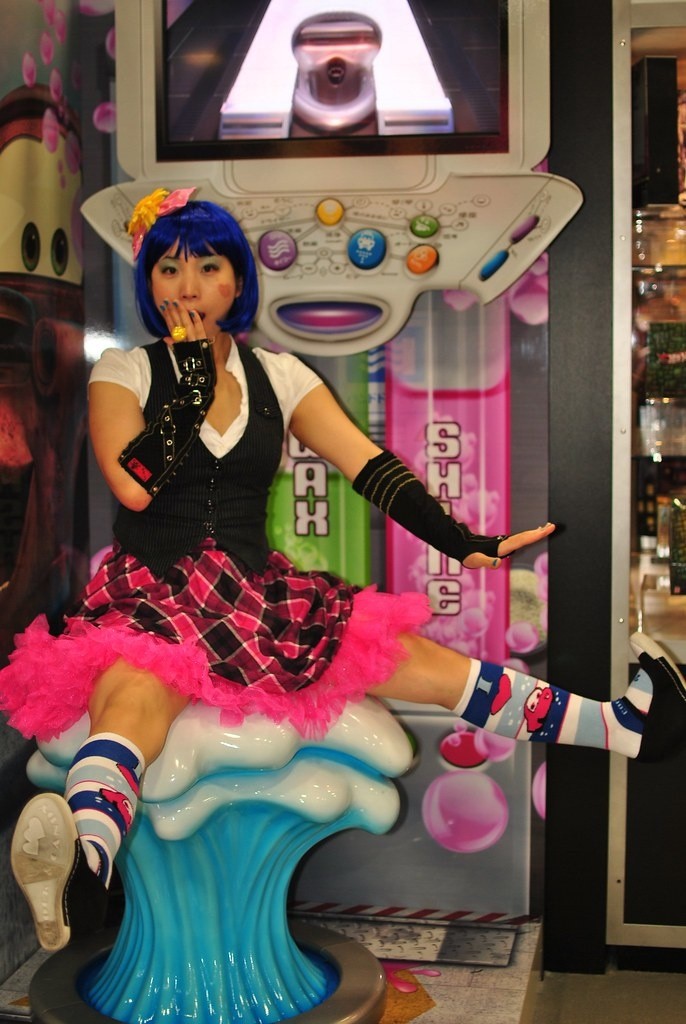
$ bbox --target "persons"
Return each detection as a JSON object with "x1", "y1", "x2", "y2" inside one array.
[{"x1": 1, "y1": 185, "x2": 685, "y2": 954}]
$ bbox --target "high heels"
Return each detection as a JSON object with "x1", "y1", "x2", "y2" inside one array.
[
  {"x1": 624, "y1": 632, "x2": 686, "y2": 763},
  {"x1": 10, "y1": 792, "x2": 110, "y2": 952}
]
